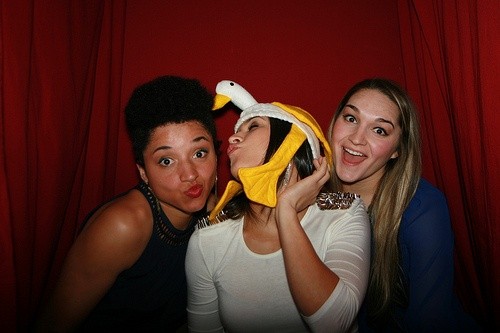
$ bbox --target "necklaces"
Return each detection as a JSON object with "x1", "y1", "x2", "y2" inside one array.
[{"x1": 139, "y1": 183, "x2": 200, "y2": 247}]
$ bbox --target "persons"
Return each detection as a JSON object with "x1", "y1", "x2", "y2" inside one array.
[
  {"x1": 327, "y1": 78, "x2": 458, "y2": 333},
  {"x1": 41, "y1": 74, "x2": 225, "y2": 333},
  {"x1": 182, "y1": 80, "x2": 373, "y2": 333}
]
